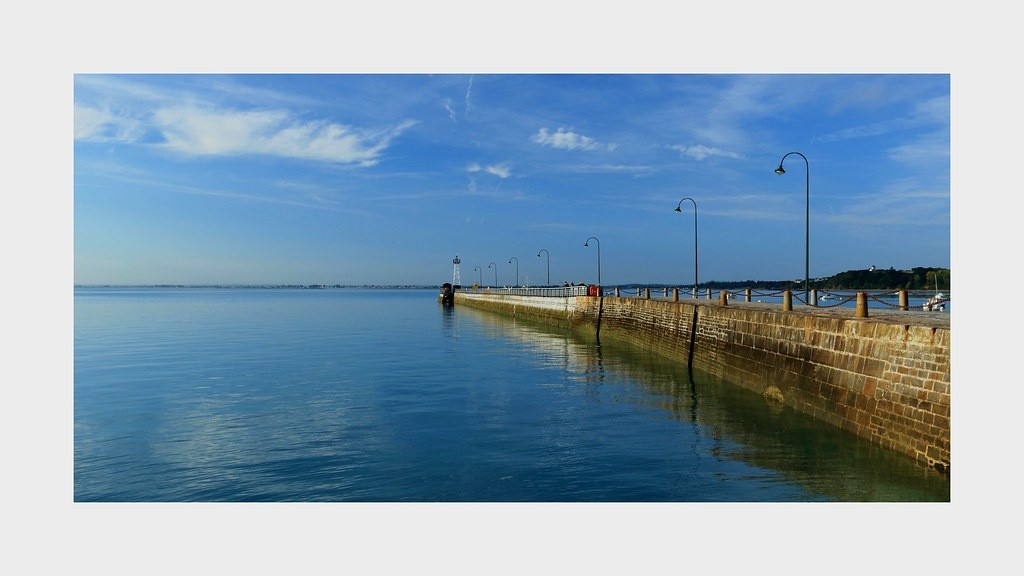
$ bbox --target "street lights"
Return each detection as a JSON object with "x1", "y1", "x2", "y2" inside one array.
[
  {"x1": 673, "y1": 197, "x2": 699, "y2": 298},
  {"x1": 583, "y1": 237, "x2": 601, "y2": 295},
  {"x1": 537, "y1": 248, "x2": 550, "y2": 288},
  {"x1": 776, "y1": 151, "x2": 810, "y2": 305},
  {"x1": 474, "y1": 266, "x2": 482, "y2": 289},
  {"x1": 488, "y1": 263, "x2": 497, "y2": 287},
  {"x1": 508, "y1": 257, "x2": 519, "y2": 286}
]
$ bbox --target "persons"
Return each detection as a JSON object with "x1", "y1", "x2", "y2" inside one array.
[{"x1": 564, "y1": 281, "x2": 570, "y2": 287}]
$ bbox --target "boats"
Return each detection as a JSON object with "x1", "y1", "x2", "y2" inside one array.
[
  {"x1": 923, "y1": 274, "x2": 947, "y2": 313},
  {"x1": 820, "y1": 294, "x2": 835, "y2": 299}
]
